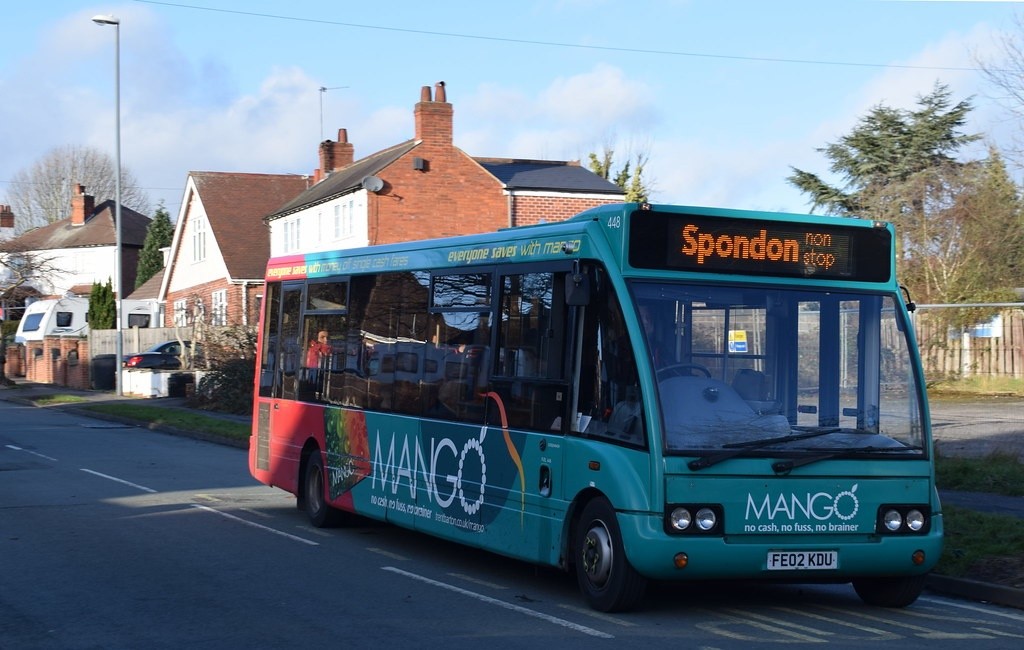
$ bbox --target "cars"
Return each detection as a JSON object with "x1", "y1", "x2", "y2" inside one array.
[{"x1": 122, "y1": 338, "x2": 243, "y2": 370}]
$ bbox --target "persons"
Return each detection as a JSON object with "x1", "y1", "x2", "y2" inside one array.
[
  {"x1": 605, "y1": 304, "x2": 667, "y2": 383},
  {"x1": 303, "y1": 329, "x2": 344, "y2": 370}
]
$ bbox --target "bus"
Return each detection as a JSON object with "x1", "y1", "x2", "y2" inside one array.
[{"x1": 246, "y1": 202, "x2": 949, "y2": 611}]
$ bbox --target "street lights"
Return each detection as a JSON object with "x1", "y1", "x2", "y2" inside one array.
[{"x1": 91, "y1": 12, "x2": 124, "y2": 394}]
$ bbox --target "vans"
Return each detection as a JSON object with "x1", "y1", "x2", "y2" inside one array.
[{"x1": 13, "y1": 295, "x2": 158, "y2": 343}]
